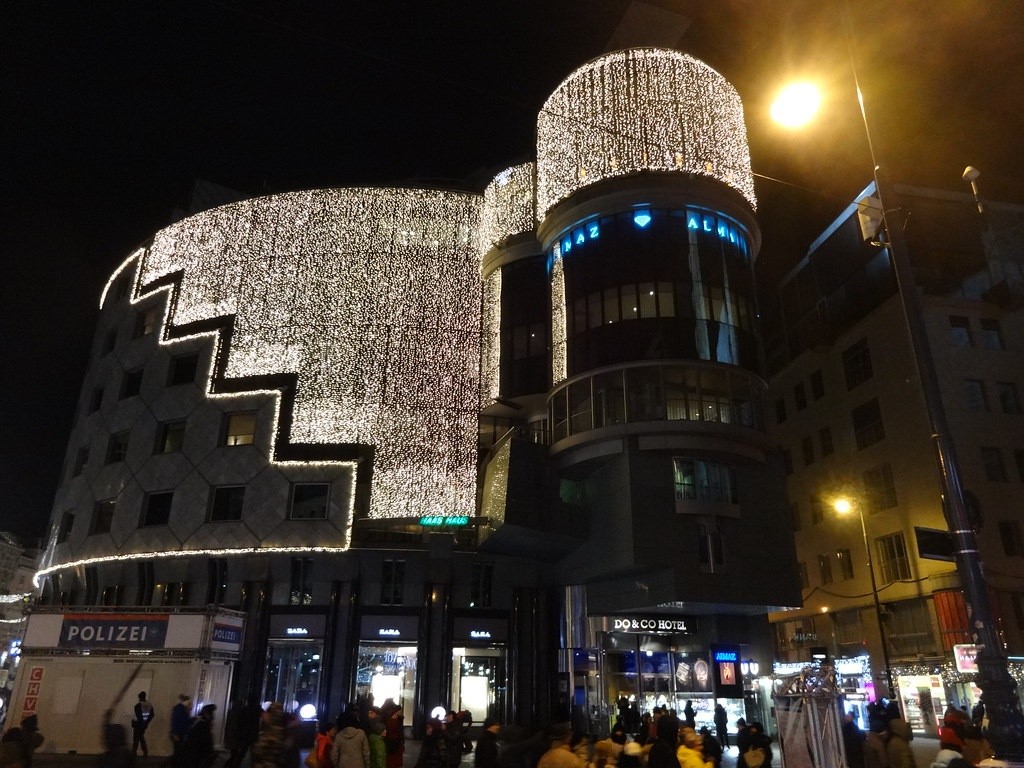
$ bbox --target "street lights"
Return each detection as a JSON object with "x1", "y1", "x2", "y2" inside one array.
[
  {"x1": 764, "y1": 57, "x2": 1023, "y2": 762},
  {"x1": 833, "y1": 498, "x2": 896, "y2": 702}
]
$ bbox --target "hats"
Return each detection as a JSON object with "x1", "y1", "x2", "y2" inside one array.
[
  {"x1": 546, "y1": 723, "x2": 575, "y2": 740},
  {"x1": 737, "y1": 718, "x2": 746, "y2": 726},
  {"x1": 940, "y1": 727, "x2": 963, "y2": 745},
  {"x1": 372, "y1": 722, "x2": 386, "y2": 735},
  {"x1": 368, "y1": 706, "x2": 381, "y2": 716},
  {"x1": 484, "y1": 717, "x2": 499, "y2": 731},
  {"x1": 624, "y1": 742, "x2": 645, "y2": 755},
  {"x1": 426, "y1": 718, "x2": 442, "y2": 729}
]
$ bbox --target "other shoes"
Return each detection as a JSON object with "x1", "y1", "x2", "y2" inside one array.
[
  {"x1": 142, "y1": 751, "x2": 148, "y2": 757},
  {"x1": 132, "y1": 751, "x2": 138, "y2": 756}
]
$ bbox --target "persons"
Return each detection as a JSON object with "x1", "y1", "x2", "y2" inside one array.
[
  {"x1": 843, "y1": 699, "x2": 986, "y2": 768},
  {"x1": 128, "y1": 684, "x2": 775, "y2": 768}
]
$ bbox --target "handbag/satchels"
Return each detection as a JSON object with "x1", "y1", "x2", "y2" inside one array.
[
  {"x1": 743, "y1": 747, "x2": 766, "y2": 768},
  {"x1": 304, "y1": 742, "x2": 320, "y2": 768}
]
